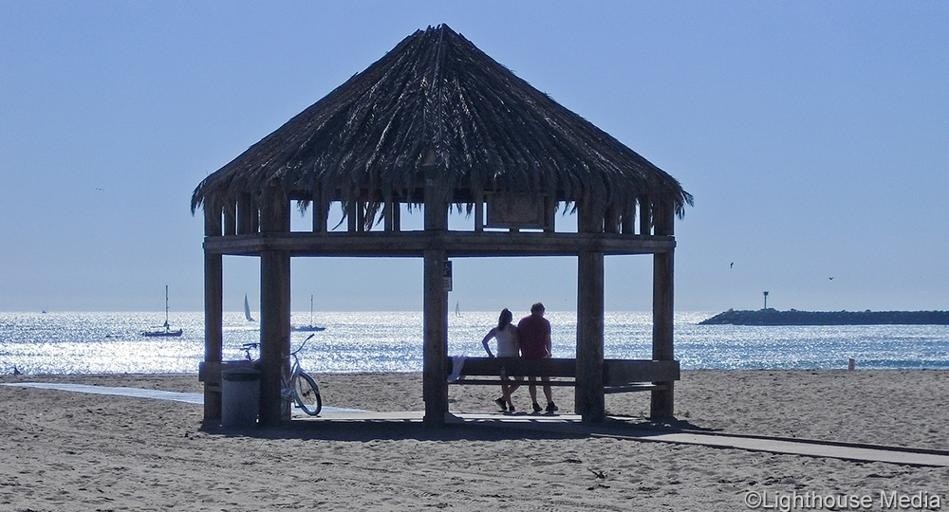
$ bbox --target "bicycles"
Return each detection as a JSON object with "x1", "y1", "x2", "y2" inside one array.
[
  {"x1": 279, "y1": 333, "x2": 324, "y2": 419},
  {"x1": 238, "y1": 341, "x2": 261, "y2": 361}
]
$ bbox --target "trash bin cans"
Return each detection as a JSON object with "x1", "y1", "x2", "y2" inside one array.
[{"x1": 220, "y1": 366, "x2": 266, "y2": 430}]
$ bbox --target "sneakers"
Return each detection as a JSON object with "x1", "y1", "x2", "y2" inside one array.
[
  {"x1": 532, "y1": 401, "x2": 559, "y2": 411},
  {"x1": 495, "y1": 397, "x2": 515, "y2": 412}
]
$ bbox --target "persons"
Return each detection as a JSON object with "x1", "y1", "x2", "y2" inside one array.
[
  {"x1": 518, "y1": 302, "x2": 559, "y2": 411},
  {"x1": 481, "y1": 308, "x2": 524, "y2": 412}
]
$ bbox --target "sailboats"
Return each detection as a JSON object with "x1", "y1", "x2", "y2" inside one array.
[
  {"x1": 243, "y1": 293, "x2": 256, "y2": 321},
  {"x1": 291, "y1": 295, "x2": 327, "y2": 331},
  {"x1": 455, "y1": 301, "x2": 463, "y2": 318},
  {"x1": 143, "y1": 284, "x2": 184, "y2": 337}
]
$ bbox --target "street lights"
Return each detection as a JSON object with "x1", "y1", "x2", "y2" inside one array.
[{"x1": 763, "y1": 292, "x2": 769, "y2": 308}]
